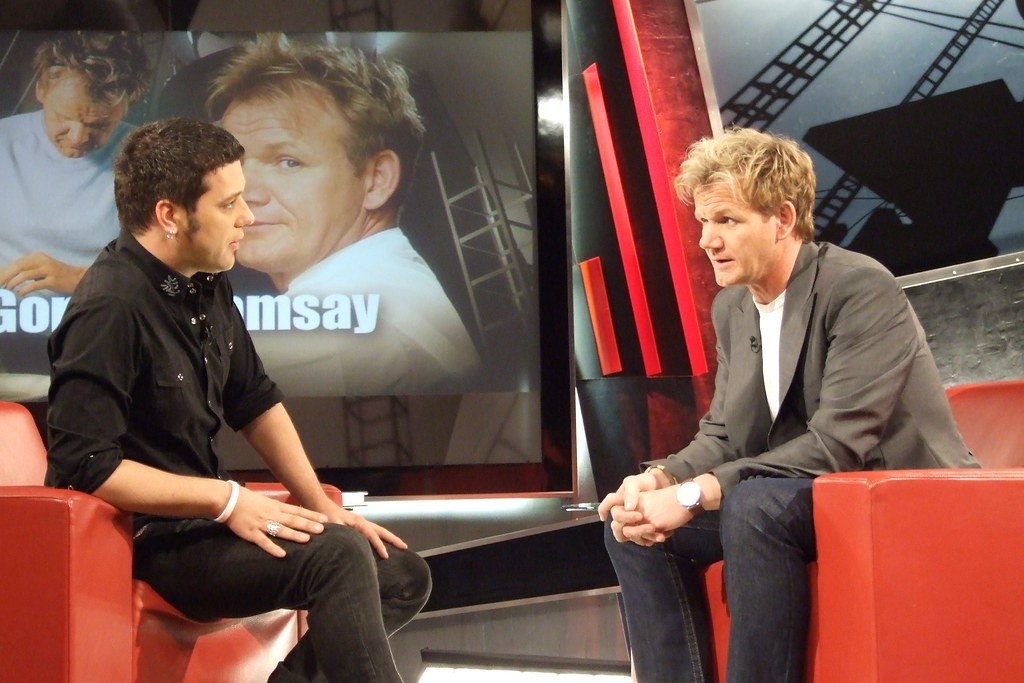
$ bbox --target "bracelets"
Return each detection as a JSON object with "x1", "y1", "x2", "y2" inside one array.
[{"x1": 214, "y1": 480, "x2": 238, "y2": 523}]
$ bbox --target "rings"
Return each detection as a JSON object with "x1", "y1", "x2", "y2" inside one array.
[{"x1": 268, "y1": 522, "x2": 281, "y2": 536}]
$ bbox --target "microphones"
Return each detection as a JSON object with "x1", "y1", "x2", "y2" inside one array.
[
  {"x1": 750, "y1": 336, "x2": 756, "y2": 342},
  {"x1": 202, "y1": 326, "x2": 210, "y2": 339}
]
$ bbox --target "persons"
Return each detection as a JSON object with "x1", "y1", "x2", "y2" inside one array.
[
  {"x1": 598, "y1": 128, "x2": 981, "y2": 683},
  {"x1": 644, "y1": 464, "x2": 675, "y2": 485},
  {"x1": 46, "y1": 119, "x2": 432, "y2": 683},
  {"x1": 1, "y1": 34, "x2": 484, "y2": 396}
]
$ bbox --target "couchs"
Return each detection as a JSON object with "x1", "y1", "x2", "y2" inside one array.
[
  {"x1": 0, "y1": 401, "x2": 342, "y2": 683},
  {"x1": 705, "y1": 382, "x2": 1024, "y2": 683}
]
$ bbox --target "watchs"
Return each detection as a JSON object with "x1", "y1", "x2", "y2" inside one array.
[{"x1": 679, "y1": 478, "x2": 704, "y2": 517}]
